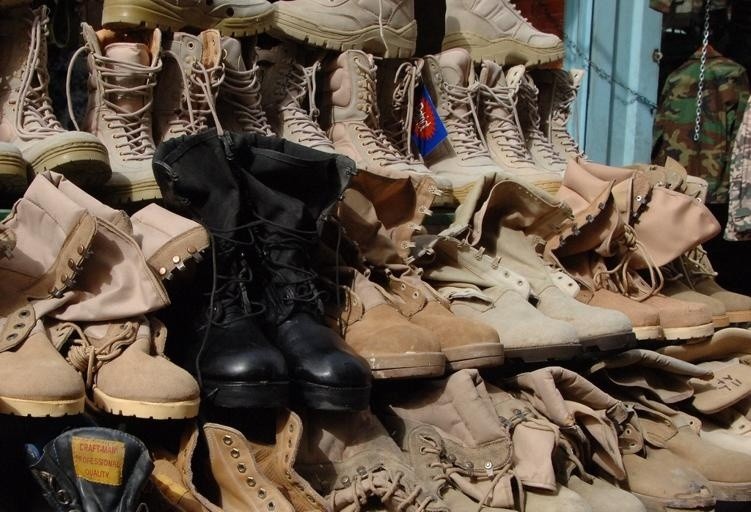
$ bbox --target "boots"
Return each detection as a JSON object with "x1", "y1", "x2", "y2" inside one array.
[{"x1": 0, "y1": 0, "x2": 751, "y2": 512}]
